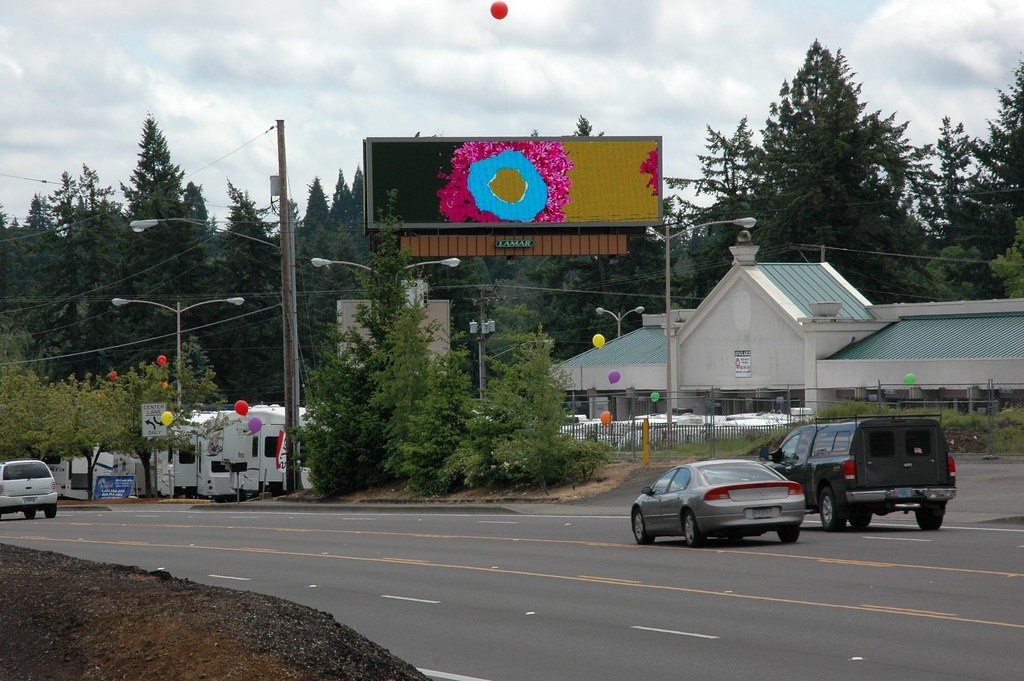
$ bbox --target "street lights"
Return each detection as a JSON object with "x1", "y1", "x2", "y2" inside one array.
[
  {"x1": 129, "y1": 218, "x2": 303, "y2": 490},
  {"x1": 666, "y1": 217, "x2": 756, "y2": 431},
  {"x1": 596, "y1": 306, "x2": 645, "y2": 338},
  {"x1": 111, "y1": 298, "x2": 245, "y2": 409}
]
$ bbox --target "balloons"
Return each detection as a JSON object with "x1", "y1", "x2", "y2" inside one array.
[
  {"x1": 157, "y1": 355, "x2": 167, "y2": 366},
  {"x1": 235, "y1": 400, "x2": 248, "y2": 416},
  {"x1": 651, "y1": 392, "x2": 660, "y2": 403},
  {"x1": 248, "y1": 418, "x2": 262, "y2": 433},
  {"x1": 600, "y1": 411, "x2": 613, "y2": 426},
  {"x1": 593, "y1": 334, "x2": 605, "y2": 348},
  {"x1": 609, "y1": 371, "x2": 620, "y2": 384},
  {"x1": 490, "y1": 1, "x2": 508, "y2": 20},
  {"x1": 904, "y1": 373, "x2": 917, "y2": 386},
  {"x1": 161, "y1": 412, "x2": 173, "y2": 426},
  {"x1": 110, "y1": 371, "x2": 117, "y2": 380}
]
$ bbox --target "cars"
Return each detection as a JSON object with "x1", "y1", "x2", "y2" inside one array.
[
  {"x1": 631, "y1": 459, "x2": 806, "y2": 548},
  {"x1": 0, "y1": 460, "x2": 57, "y2": 519}
]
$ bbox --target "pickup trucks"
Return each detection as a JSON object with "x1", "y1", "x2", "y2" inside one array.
[{"x1": 759, "y1": 412, "x2": 958, "y2": 533}]
